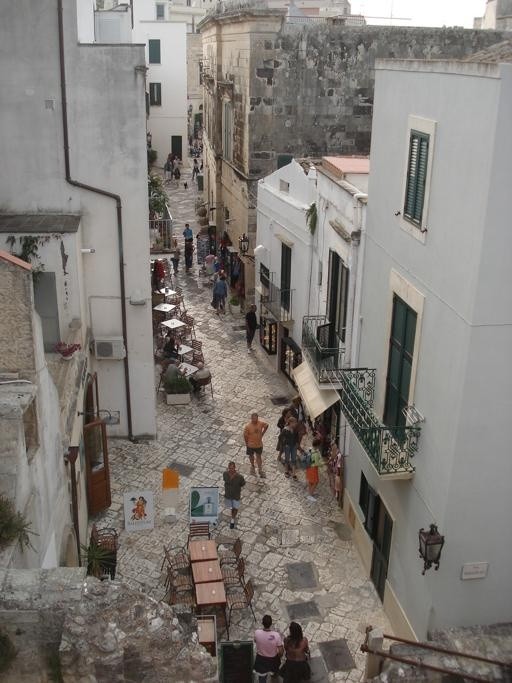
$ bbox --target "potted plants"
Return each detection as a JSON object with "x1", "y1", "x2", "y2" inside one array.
[{"x1": 148, "y1": 172, "x2": 170, "y2": 236}]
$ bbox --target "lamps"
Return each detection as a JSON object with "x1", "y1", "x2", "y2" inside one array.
[
  {"x1": 78, "y1": 409, "x2": 120, "y2": 425},
  {"x1": 147, "y1": 131, "x2": 152, "y2": 148},
  {"x1": 419, "y1": 524, "x2": 445, "y2": 575},
  {"x1": 239, "y1": 233, "x2": 255, "y2": 263}
]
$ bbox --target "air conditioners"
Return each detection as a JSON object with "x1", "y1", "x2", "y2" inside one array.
[{"x1": 95, "y1": 336, "x2": 124, "y2": 360}]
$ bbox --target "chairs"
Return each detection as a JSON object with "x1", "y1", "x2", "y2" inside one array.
[
  {"x1": 161, "y1": 522, "x2": 257, "y2": 641},
  {"x1": 152, "y1": 285, "x2": 213, "y2": 401}
]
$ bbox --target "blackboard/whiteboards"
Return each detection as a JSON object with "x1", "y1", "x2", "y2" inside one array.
[{"x1": 221, "y1": 643, "x2": 254, "y2": 682}]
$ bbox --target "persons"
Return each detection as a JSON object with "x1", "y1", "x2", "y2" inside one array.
[
  {"x1": 278, "y1": 621, "x2": 311, "y2": 683},
  {"x1": 245, "y1": 304, "x2": 257, "y2": 354},
  {"x1": 162, "y1": 153, "x2": 181, "y2": 189},
  {"x1": 275, "y1": 396, "x2": 330, "y2": 503},
  {"x1": 252, "y1": 614, "x2": 284, "y2": 683},
  {"x1": 243, "y1": 413, "x2": 269, "y2": 478},
  {"x1": 187, "y1": 127, "x2": 203, "y2": 181},
  {"x1": 222, "y1": 461, "x2": 246, "y2": 529},
  {"x1": 151, "y1": 224, "x2": 244, "y2": 395}
]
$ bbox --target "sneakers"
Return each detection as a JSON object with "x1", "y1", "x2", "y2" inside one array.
[
  {"x1": 247, "y1": 347, "x2": 256, "y2": 354},
  {"x1": 230, "y1": 519, "x2": 235, "y2": 529}
]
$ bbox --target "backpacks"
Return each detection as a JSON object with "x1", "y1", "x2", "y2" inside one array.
[{"x1": 300, "y1": 449, "x2": 315, "y2": 469}]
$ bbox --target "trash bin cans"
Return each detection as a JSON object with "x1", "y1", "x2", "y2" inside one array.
[{"x1": 198, "y1": 174, "x2": 204, "y2": 191}]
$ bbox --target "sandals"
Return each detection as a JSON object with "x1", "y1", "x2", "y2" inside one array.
[{"x1": 284, "y1": 471, "x2": 298, "y2": 481}]
